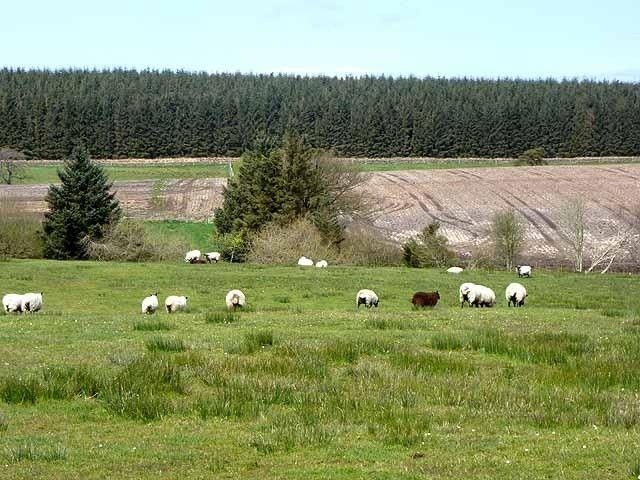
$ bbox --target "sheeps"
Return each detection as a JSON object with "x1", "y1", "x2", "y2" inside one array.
[
  {"x1": 1, "y1": 290, "x2": 44, "y2": 314},
  {"x1": 459, "y1": 282, "x2": 496, "y2": 309},
  {"x1": 165, "y1": 294, "x2": 189, "y2": 314},
  {"x1": 514, "y1": 265, "x2": 532, "y2": 278},
  {"x1": 356, "y1": 288, "x2": 379, "y2": 309},
  {"x1": 505, "y1": 282, "x2": 528, "y2": 307},
  {"x1": 140, "y1": 292, "x2": 159, "y2": 314},
  {"x1": 411, "y1": 290, "x2": 441, "y2": 310},
  {"x1": 297, "y1": 254, "x2": 327, "y2": 268},
  {"x1": 225, "y1": 289, "x2": 246, "y2": 310},
  {"x1": 183, "y1": 249, "x2": 221, "y2": 264},
  {"x1": 446, "y1": 267, "x2": 463, "y2": 273}
]
